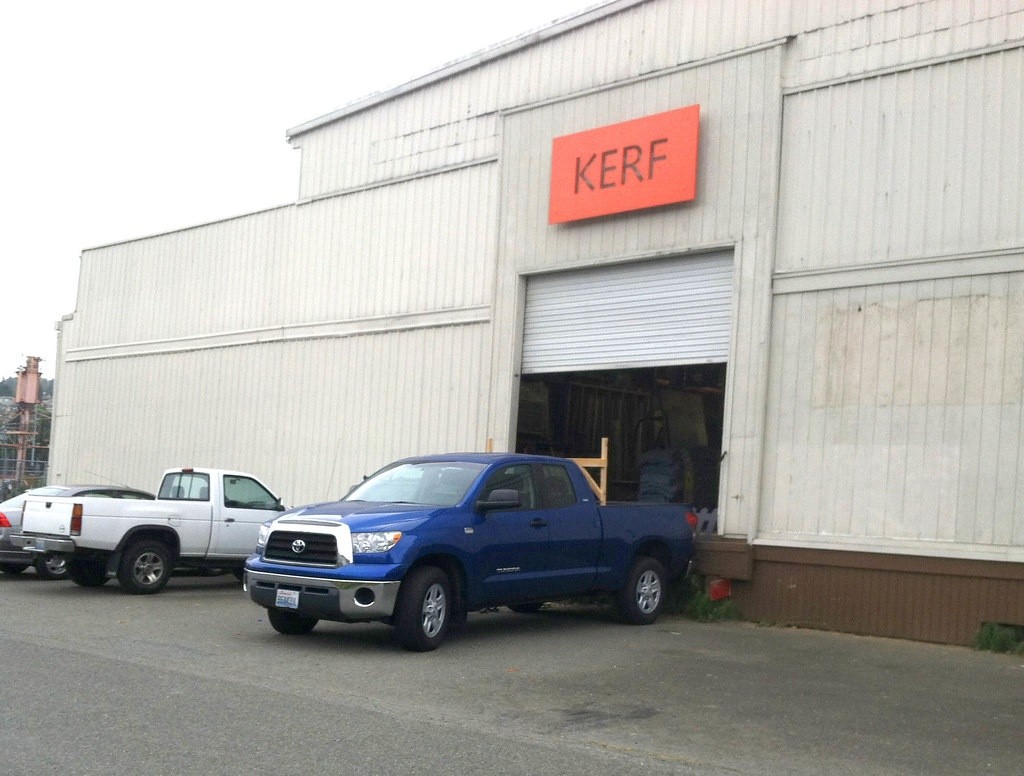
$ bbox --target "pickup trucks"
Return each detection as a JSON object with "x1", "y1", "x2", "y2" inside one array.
[
  {"x1": 7, "y1": 468, "x2": 288, "y2": 595},
  {"x1": 242, "y1": 452, "x2": 703, "y2": 652}
]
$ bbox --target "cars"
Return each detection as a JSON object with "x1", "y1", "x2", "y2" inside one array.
[{"x1": 0, "y1": 485, "x2": 157, "y2": 581}]
